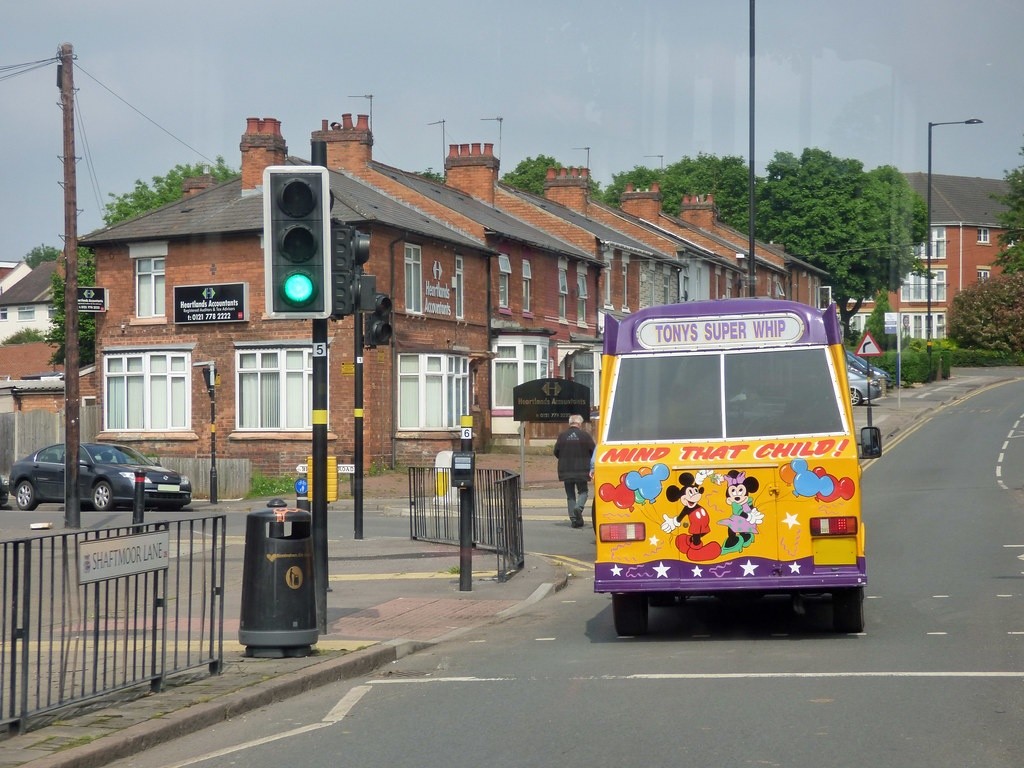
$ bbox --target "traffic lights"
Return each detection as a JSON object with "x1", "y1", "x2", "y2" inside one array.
[
  {"x1": 366, "y1": 292, "x2": 392, "y2": 347},
  {"x1": 263, "y1": 163, "x2": 332, "y2": 319},
  {"x1": 329, "y1": 217, "x2": 369, "y2": 318}
]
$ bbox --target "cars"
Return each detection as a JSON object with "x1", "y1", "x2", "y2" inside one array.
[
  {"x1": 9, "y1": 444, "x2": 191, "y2": 511},
  {"x1": 845, "y1": 367, "x2": 882, "y2": 405}
]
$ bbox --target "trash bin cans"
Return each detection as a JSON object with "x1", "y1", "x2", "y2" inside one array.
[
  {"x1": 237, "y1": 507, "x2": 319, "y2": 659},
  {"x1": 433, "y1": 450, "x2": 460, "y2": 506}
]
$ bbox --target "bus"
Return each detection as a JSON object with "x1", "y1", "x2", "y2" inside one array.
[{"x1": 595, "y1": 296, "x2": 882, "y2": 635}]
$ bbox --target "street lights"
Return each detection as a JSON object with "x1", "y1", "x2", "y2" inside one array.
[{"x1": 927, "y1": 119, "x2": 983, "y2": 357}]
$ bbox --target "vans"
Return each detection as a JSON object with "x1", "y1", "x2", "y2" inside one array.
[{"x1": 845, "y1": 349, "x2": 891, "y2": 392}]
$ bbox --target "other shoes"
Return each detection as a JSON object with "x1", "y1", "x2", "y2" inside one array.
[
  {"x1": 571, "y1": 521, "x2": 578, "y2": 528},
  {"x1": 574, "y1": 508, "x2": 583, "y2": 527}
]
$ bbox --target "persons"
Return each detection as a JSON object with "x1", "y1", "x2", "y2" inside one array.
[{"x1": 553, "y1": 416, "x2": 596, "y2": 544}]
